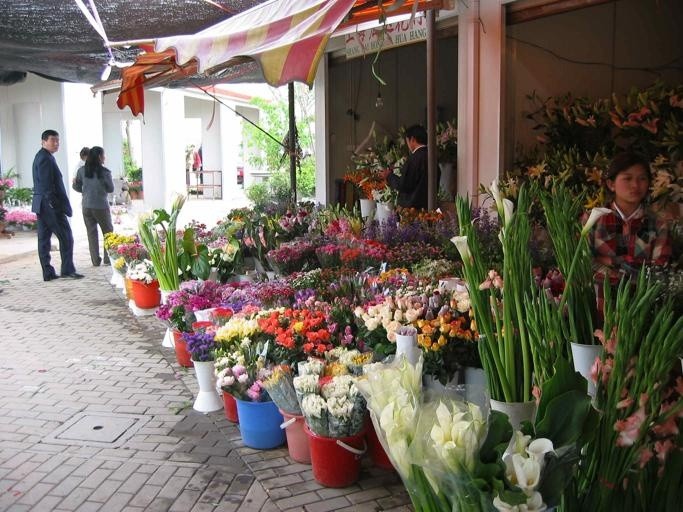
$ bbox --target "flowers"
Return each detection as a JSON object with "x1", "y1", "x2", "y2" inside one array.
[{"x1": 0, "y1": 164, "x2": 37, "y2": 239}]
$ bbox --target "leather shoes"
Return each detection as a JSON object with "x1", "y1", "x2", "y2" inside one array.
[
  {"x1": 43, "y1": 275, "x2": 59, "y2": 281},
  {"x1": 60, "y1": 273, "x2": 84, "y2": 279}
]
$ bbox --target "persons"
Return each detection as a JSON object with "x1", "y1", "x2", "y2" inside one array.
[
  {"x1": 198, "y1": 142, "x2": 203, "y2": 184},
  {"x1": 31, "y1": 129, "x2": 85, "y2": 281},
  {"x1": 72, "y1": 146, "x2": 114, "y2": 267},
  {"x1": 378, "y1": 123, "x2": 442, "y2": 212},
  {"x1": 582, "y1": 152, "x2": 675, "y2": 323},
  {"x1": 78, "y1": 146, "x2": 93, "y2": 165},
  {"x1": 186, "y1": 144, "x2": 202, "y2": 172}
]
